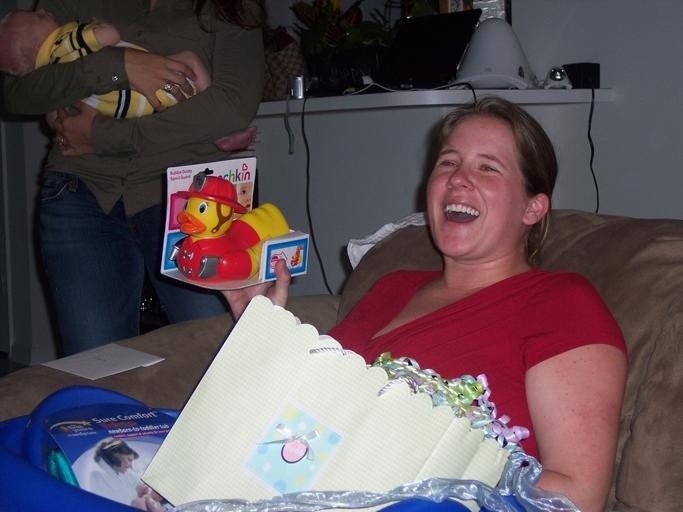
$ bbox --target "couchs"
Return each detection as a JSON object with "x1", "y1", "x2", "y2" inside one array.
[{"x1": 1, "y1": 210, "x2": 680, "y2": 511}]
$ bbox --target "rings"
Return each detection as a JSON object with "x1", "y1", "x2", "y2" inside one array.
[
  {"x1": 162, "y1": 82, "x2": 172, "y2": 93},
  {"x1": 53, "y1": 130, "x2": 66, "y2": 147}
]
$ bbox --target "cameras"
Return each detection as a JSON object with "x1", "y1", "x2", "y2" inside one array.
[{"x1": 287, "y1": 72, "x2": 305, "y2": 99}]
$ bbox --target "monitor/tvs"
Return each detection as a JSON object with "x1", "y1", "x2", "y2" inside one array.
[{"x1": 391, "y1": 8, "x2": 482, "y2": 86}]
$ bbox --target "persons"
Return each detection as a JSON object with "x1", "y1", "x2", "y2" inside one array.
[
  {"x1": 216, "y1": 96, "x2": 630, "y2": 512},
  {"x1": 236, "y1": 182, "x2": 252, "y2": 214},
  {"x1": 0, "y1": 0, "x2": 282, "y2": 357},
  {"x1": 91, "y1": 437, "x2": 139, "y2": 506},
  {"x1": 0, "y1": 9, "x2": 262, "y2": 155},
  {"x1": 130, "y1": 480, "x2": 167, "y2": 511}
]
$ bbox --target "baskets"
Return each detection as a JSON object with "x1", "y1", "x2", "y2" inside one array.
[{"x1": 264, "y1": 42, "x2": 303, "y2": 101}]
